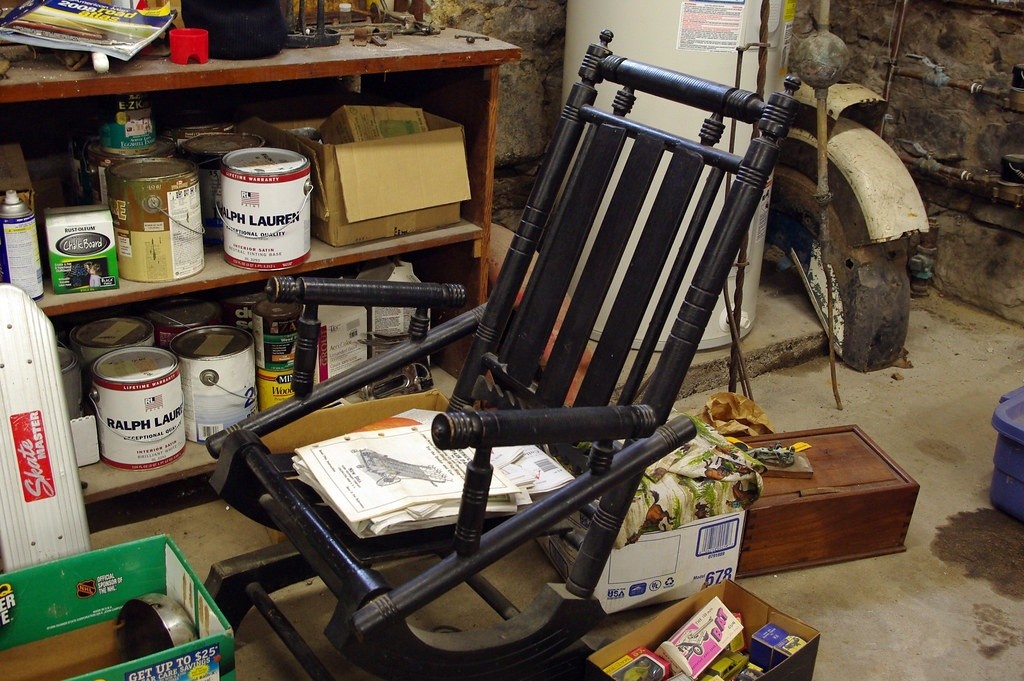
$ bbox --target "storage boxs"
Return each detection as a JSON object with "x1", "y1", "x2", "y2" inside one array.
[
  {"x1": 259, "y1": 387, "x2": 449, "y2": 545},
  {"x1": 0, "y1": 533, "x2": 236, "y2": 681},
  {"x1": 238, "y1": 101, "x2": 472, "y2": 246},
  {"x1": 302, "y1": 304, "x2": 368, "y2": 384},
  {"x1": 356, "y1": 259, "x2": 431, "y2": 368},
  {"x1": 44, "y1": 204, "x2": 119, "y2": 293},
  {"x1": 536, "y1": 403, "x2": 768, "y2": 614},
  {"x1": 732, "y1": 423, "x2": 920, "y2": 579},
  {"x1": 585, "y1": 579, "x2": 820, "y2": 681},
  {"x1": 0, "y1": 142, "x2": 35, "y2": 210},
  {"x1": 990, "y1": 385, "x2": 1024, "y2": 526}
]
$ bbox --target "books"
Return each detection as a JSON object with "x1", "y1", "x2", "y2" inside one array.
[
  {"x1": 293, "y1": 402, "x2": 575, "y2": 538},
  {"x1": 0, "y1": 0, "x2": 174, "y2": 60}
]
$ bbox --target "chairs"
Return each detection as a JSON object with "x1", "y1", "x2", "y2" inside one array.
[{"x1": 204, "y1": 28, "x2": 803, "y2": 681}]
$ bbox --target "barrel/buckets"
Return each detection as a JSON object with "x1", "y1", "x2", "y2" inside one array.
[
  {"x1": 55, "y1": 290, "x2": 309, "y2": 472},
  {"x1": 70, "y1": 92, "x2": 314, "y2": 283}
]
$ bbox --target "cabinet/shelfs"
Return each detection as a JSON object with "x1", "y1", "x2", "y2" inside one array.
[{"x1": 0, "y1": 25, "x2": 524, "y2": 505}]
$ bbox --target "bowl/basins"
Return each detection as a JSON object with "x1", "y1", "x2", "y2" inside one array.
[{"x1": 116, "y1": 593, "x2": 197, "y2": 658}]
